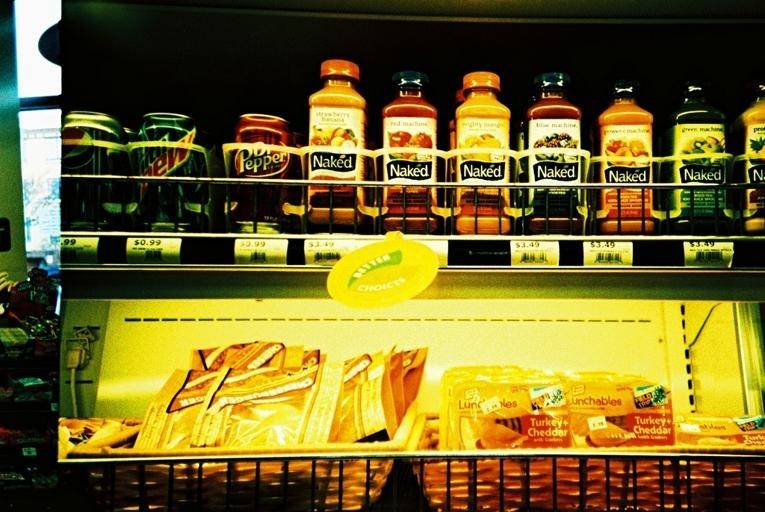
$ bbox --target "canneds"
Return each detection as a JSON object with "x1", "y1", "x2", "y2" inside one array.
[
  {"x1": 235, "y1": 113, "x2": 290, "y2": 222},
  {"x1": 139, "y1": 112, "x2": 202, "y2": 231},
  {"x1": 63, "y1": 110, "x2": 125, "y2": 231}
]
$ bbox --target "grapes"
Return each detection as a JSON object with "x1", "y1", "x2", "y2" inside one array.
[{"x1": 535, "y1": 133, "x2": 576, "y2": 148}]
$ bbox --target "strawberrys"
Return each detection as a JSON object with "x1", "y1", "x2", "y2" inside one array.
[{"x1": 388, "y1": 131, "x2": 432, "y2": 148}]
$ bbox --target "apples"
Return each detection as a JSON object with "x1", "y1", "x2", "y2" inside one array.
[
  {"x1": 312, "y1": 127, "x2": 354, "y2": 147},
  {"x1": 701, "y1": 143, "x2": 720, "y2": 153}
]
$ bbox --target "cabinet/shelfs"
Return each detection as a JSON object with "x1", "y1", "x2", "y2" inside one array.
[{"x1": 56, "y1": 173, "x2": 765, "y2": 512}]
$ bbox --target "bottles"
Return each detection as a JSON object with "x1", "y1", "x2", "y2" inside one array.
[
  {"x1": 528, "y1": 71, "x2": 582, "y2": 236},
  {"x1": 741, "y1": 82, "x2": 765, "y2": 232},
  {"x1": 673, "y1": 79, "x2": 727, "y2": 222},
  {"x1": 598, "y1": 79, "x2": 655, "y2": 234},
  {"x1": 380, "y1": 68, "x2": 438, "y2": 232},
  {"x1": 452, "y1": 71, "x2": 513, "y2": 235},
  {"x1": 307, "y1": 58, "x2": 367, "y2": 226}
]
